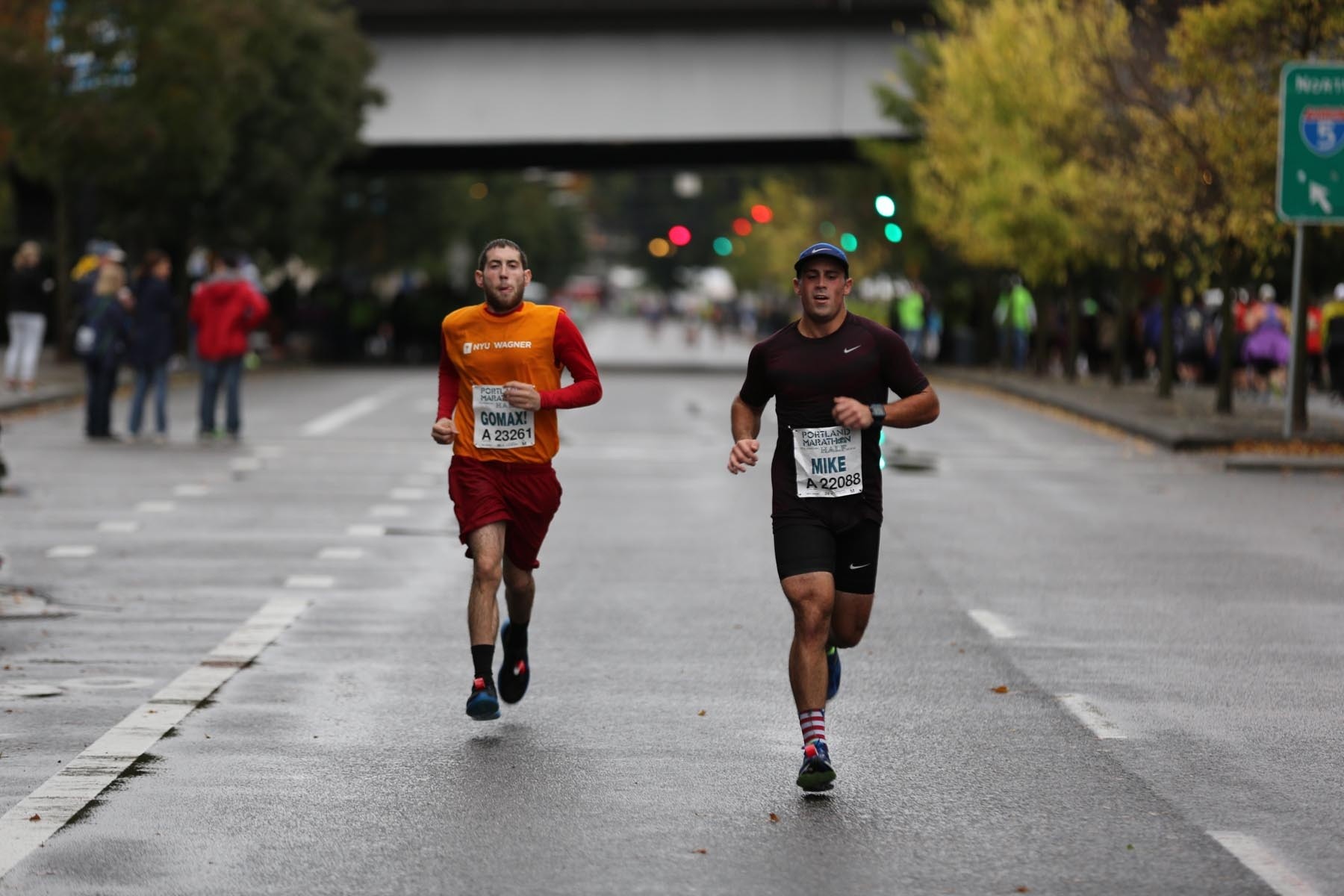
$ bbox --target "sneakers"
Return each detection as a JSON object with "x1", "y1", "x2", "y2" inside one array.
[
  {"x1": 825, "y1": 647, "x2": 841, "y2": 701},
  {"x1": 796, "y1": 737, "x2": 837, "y2": 792},
  {"x1": 465, "y1": 677, "x2": 500, "y2": 721},
  {"x1": 498, "y1": 618, "x2": 529, "y2": 704}
]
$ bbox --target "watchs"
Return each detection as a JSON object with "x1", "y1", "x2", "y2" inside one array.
[{"x1": 867, "y1": 400, "x2": 886, "y2": 427}]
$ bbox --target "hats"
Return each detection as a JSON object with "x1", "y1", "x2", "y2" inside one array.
[{"x1": 793, "y1": 242, "x2": 850, "y2": 271}]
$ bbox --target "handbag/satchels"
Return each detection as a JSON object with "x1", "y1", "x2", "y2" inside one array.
[{"x1": 73, "y1": 324, "x2": 99, "y2": 357}]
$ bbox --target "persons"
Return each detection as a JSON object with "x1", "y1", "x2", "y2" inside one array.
[
  {"x1": 0, "y1": 237, "x2": 1344, "y2": 481},
  {"x1": 728, "y1": 245, "x2": 938, "y2": 792},
  {"x1": 431, "y1": 239, "x2": 604, "y2": 721}
]
